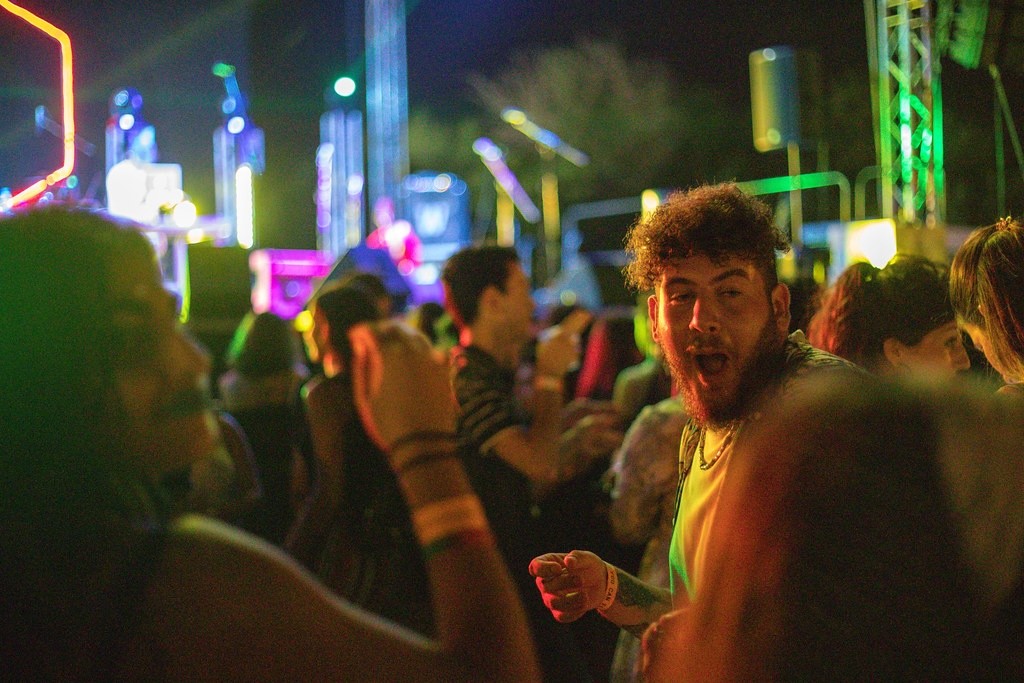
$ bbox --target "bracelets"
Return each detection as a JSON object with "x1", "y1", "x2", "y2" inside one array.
[
  {"x1": 386, "y1": 427, "x2": 461, "y2": 459},
  {"x1": 596, "y1": 559, "x2": 617, "y2": 611},
  {"x1": 393, "y1": 450, "x2": 459, "y2": 482}
]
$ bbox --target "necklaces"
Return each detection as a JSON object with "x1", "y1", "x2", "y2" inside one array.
[{"x1": 700, "y1": 414, "x2": 735, "y2": 470}]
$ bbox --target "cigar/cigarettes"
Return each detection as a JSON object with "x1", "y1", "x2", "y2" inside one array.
[{"x1": 541, "y1": 566, "x2": 568, "y2": 584}]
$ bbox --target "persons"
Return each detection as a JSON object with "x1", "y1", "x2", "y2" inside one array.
[{"x1": 0, "y1": 182, "x2": 1024, "y2": 683}]
